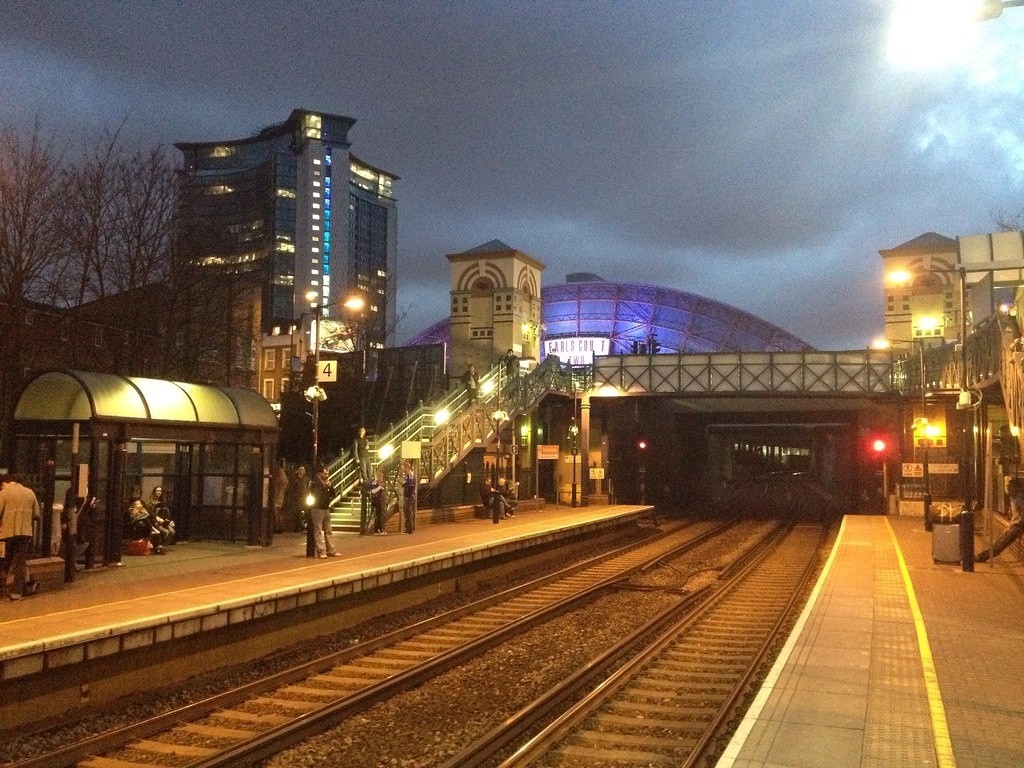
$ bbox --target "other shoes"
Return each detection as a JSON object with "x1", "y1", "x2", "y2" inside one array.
[
  {"x1": 374, "y1": 529, "x2": 388, "y2": 536},
  {"x1": 330, "y1": 553, "x2": 343, "y2": 556},
  {"x1": 400, "y1": 530, "x2": 413, "y2": 535},
  {"x1": 501, "y1": 506, "x2": 517, "y2": 520},
  {"x1": 10, "y1": 590, "x2": 23, "y2": 601},
  {"x1": 155, "y1": 549, "x2": 165, "y2": 555},
  {"x1": 317, "y1": 554, "x2": 328, "y2": 559}
]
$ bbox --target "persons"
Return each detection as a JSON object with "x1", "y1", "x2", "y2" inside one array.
[
  {"x1": 124, "y1": 486, "x2": 175, "y2": 555},
  {"x1": 504, "y1": 349, "x2": 520, "y2": 380},
  {"x1": 61, "y1": 485, "x2": 99, "y2": 570},
  {"x1": 973, "y1": 479, "x2": 1024, "y2": 563},
  {"x1": 401, "y1": 462, "x2": 416, "y2": 534},
  {"x1": 353, "y1": 427, "x2": 373, "y2": 482},
  {"x1": 461, "y1": 364, "x2": 481, "y2": 406},
  {"x1": 312, "y1": 467, "x2": 343, "y2": 559},
  {"x1": 478, "y1": 477, "x2": 519, "y2": 519},
  {"x1": 370, "y1": 471, "x2": 387, "y2": 536},
  {"x1": 274, "y1": 468, "x2": 288, "y2": 510},
  {"x1": 289, "y1": 465, "x2": 310, "y2": 533},
  {"x1": 0, "y1": 474, "x2": 41, "y2": 602}
]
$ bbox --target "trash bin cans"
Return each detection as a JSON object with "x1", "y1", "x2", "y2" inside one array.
[{"x1": 931, "y1": 521, "x2": 963, "y2": 566}]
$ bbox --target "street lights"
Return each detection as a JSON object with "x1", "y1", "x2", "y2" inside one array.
[
  {"x1": 871, "y1": 337, "x2": 933, "y2": 532},
  {"x1": 489, "y1": 409, "x2": 509, "y2": 524},
  {"x1": 305, "y1": 297, "x2": 365, "y2": 558},
  {"x1": 886, "y1": 266, "x2": 976, "y2": 573}
]
